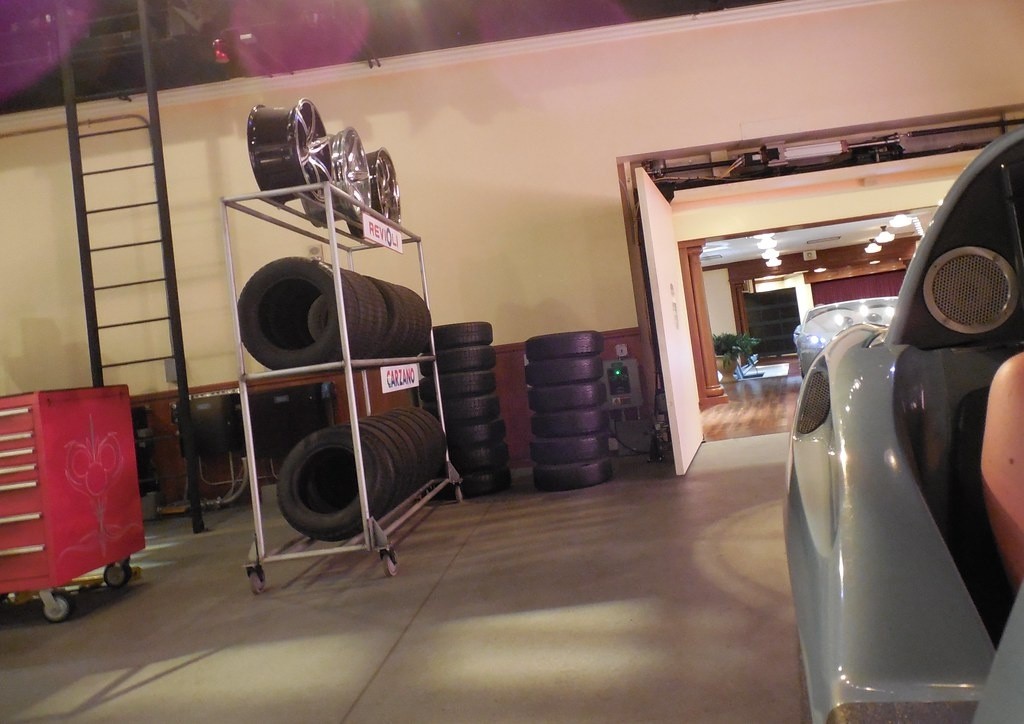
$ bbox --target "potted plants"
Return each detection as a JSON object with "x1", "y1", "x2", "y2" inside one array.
[{"x1": 713, "y1": 333, "x2": 761, "y2": 384}]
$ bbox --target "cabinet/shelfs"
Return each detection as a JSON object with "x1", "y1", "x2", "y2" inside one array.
[{"x1": 221, "y1": 180, "x2": 463, "y2": 593}]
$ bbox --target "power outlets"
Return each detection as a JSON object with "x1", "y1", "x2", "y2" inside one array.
[{"x1": 612, "y1": 397, "x2": 622, "y2": 407}]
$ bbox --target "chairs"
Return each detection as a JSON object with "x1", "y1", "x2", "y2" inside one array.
[{"x1": 949, "y1": 350, "x2": 1024, "y2": 639}]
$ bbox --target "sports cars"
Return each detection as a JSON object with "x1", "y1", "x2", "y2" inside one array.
[
  {"x1": 791, "y1": 295, "x2": 900, "y2": 381},
  {"x1": 779, "y1": 126, "x2": 1024, "y2": 724}
]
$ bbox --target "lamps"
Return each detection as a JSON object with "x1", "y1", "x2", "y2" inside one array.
[
  {"x1": 737, "y1": 138, "x2": 851, "y2": 166},
  {"x1": 868, "y1": 253, "x2": 880, "y2": 265},
  {"x1": 863, "y1": 238, "x2": 883, "y2": 253},
  {"x1": 812, "y1": 258, "x2": 828, "y2": 273},
  {"x1": 875, "y1": 225, "x2": 896, "y2": 244}
]
